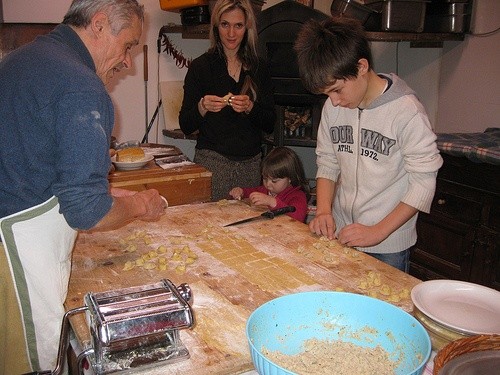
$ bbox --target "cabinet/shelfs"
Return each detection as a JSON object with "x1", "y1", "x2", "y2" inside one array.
[{"x1": 409, "y1": 152, "x2": 500, "y2": 292}]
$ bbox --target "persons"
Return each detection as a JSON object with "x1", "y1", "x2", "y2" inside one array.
[
  {"x1": 229, "y1": 147, "x2": 310, "y2": 224},
  {"x1": 0, "y1": 0, "x2": 167, "y2": 375},
  {"x1": 293, "y1": 16, "x2": 443, "y2": 274},
  {"x1": 178, "y1": 0, "x2": 276, "y2": 202}
]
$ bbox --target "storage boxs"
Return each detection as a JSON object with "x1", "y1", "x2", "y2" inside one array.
[
  {"x1": 159, "y1": 0, "x2": 212, "y2": 25},
  {"x1": 331, "y1": 0, "x2": 473, "y2": 34}
]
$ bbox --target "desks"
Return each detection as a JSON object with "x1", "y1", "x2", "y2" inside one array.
[{"x1": 113, "y1": 160, "x2": 213, "y2": 205}]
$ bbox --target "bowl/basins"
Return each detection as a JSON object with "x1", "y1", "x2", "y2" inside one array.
[{"x1": 245, "y1": 290, "x2": 432, "y2": 375}]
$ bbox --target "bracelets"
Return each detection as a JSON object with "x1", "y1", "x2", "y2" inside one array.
[
  {"x1": 200, "y1": 98, "x2": 208, "y2": 112},
  {"x1": 245, "y1": 101, "x2": 252, "y2": 112}
]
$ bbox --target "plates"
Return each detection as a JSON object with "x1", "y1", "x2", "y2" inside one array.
[
  {"x1": 110, "y1": 152, "x2": 155, "y2": 170},
  {"x1": 410, "y1": 279, "x2": 500, "y2": 335}
]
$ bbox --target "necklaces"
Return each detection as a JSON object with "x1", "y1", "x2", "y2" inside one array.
[{"x1": 228, "y1": 63, "x2": 240, "y2": 77}]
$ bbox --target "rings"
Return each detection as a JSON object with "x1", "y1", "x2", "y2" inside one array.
[{"x1": 214, "y1": 106, "x2": 216, "y2": 111}]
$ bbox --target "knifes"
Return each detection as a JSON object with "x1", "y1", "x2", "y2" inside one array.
[{"x1": 222, "y1": 205, "x2": 294, "y2": 228}]
